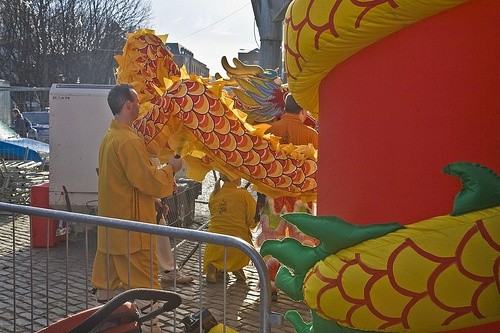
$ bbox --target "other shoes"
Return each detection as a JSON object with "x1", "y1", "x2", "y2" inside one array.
[
  {"x1": 97, "y1": 297, "x2": 113, "y2": 305},
  {"x1": 271, "y1": 291, "x2": 277, "y2": 302}
]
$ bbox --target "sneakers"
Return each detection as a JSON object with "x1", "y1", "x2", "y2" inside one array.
[
  {"x1": 233, "y1": 268, "x2": 247, "y2": 281},
  {"x1": 206, "y1": 264, "x2": 216, "y2": 284},
  {"x1": 91, "y1": 282, "x2": 123, "y2": 299},
  {"x1": 161, "y1": 269, "x2": 194, "y2": 284}
]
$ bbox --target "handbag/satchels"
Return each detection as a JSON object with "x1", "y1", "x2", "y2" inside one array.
[{"x1": 24, "y1": 118, "x2": 32, "y2": 132}]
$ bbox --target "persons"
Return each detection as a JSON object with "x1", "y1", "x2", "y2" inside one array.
[
  {"x1": 203, "y1": 172, "x2": 258, "y2": 284},
  {"x1": 151, "y1": 157, "x2": 194, "y2": 284},
  {"x1": 256, "y1": 93, "x2": 318, "y2": 302},
  {"x1": 12, "y1": 109, "x2": 27, "y2": 138},
  {"x1": 91, "y1": 83, "x2": 182, "y2": 333}
]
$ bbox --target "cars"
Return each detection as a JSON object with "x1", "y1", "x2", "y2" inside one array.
[
  {"x1": 0, "y1": 120, "x2": 52, "y2": 168},
  {"x1": 8, "y1": 111, "x2": 49, "y2": 144}
]
